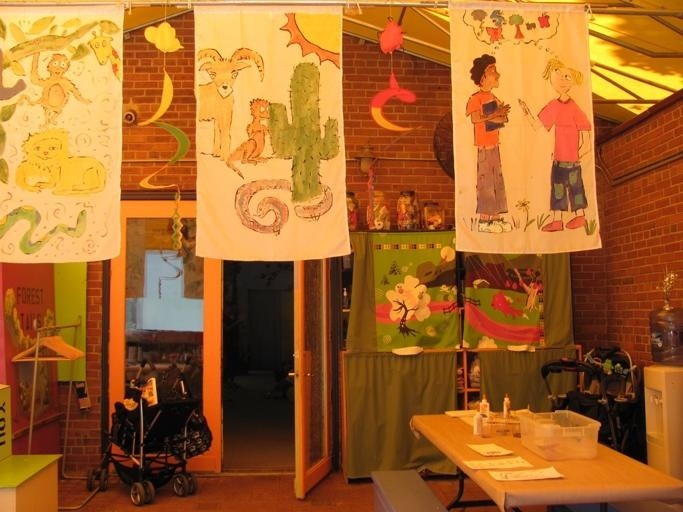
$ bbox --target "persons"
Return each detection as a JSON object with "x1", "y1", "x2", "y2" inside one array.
[
  {"x1": 466, "y1": 53, "x2": 512, "y2": 233},
  {"x1": 22, "y1": 51, "x2": 93, "y2": 126},
  {"x1": 517, "y1": 54, "x2": 592, "y2": 231}
]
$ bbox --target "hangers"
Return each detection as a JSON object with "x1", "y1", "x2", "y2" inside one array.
[{"x1": 9, "y1": 325, "x2": 86, "y2": 362}]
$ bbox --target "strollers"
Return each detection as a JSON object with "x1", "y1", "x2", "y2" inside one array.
[
  {"x1": 541, "y1": 344, "x2": 646, "y2": 458},
  {"x1": 87, "y1": 358, "x2": 212, "y2": 506}
]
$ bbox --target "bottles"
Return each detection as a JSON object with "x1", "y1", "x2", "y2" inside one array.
[
  {"x1": 473, "y1": 394, "x2": 489, "y2": 436},
  {"x1": 345, "y1": 189, "x2": 445, "y2": 232},
  {"x1": 504, "y1": 393, "x2": 511, "y2": 417}
]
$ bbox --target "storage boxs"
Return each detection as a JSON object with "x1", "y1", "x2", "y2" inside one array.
[{"x1": 511, "y1": 407, "x2": 603, "y2": 461}]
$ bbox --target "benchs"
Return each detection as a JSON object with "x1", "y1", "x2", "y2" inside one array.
[{"x1": 369, "y1": 469, "x2": 449, "y2": 512}]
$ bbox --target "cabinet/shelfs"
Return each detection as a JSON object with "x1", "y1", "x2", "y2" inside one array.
[{"x1": 335, "y1": 227, "x2": 586, "y2": 486}]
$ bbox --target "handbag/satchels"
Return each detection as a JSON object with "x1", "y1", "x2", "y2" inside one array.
[{"x1": 170, "y1": 414, "x2": 211, "y2": 460}]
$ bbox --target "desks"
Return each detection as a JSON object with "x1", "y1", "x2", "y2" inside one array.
[{"x1": 411, "y1": 410, "x2": 683, "y2": 512}]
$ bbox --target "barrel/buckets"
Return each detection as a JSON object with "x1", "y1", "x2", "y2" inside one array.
[{"x1": 648, "y1": 307, "x2": 682, "y2": 366}]
